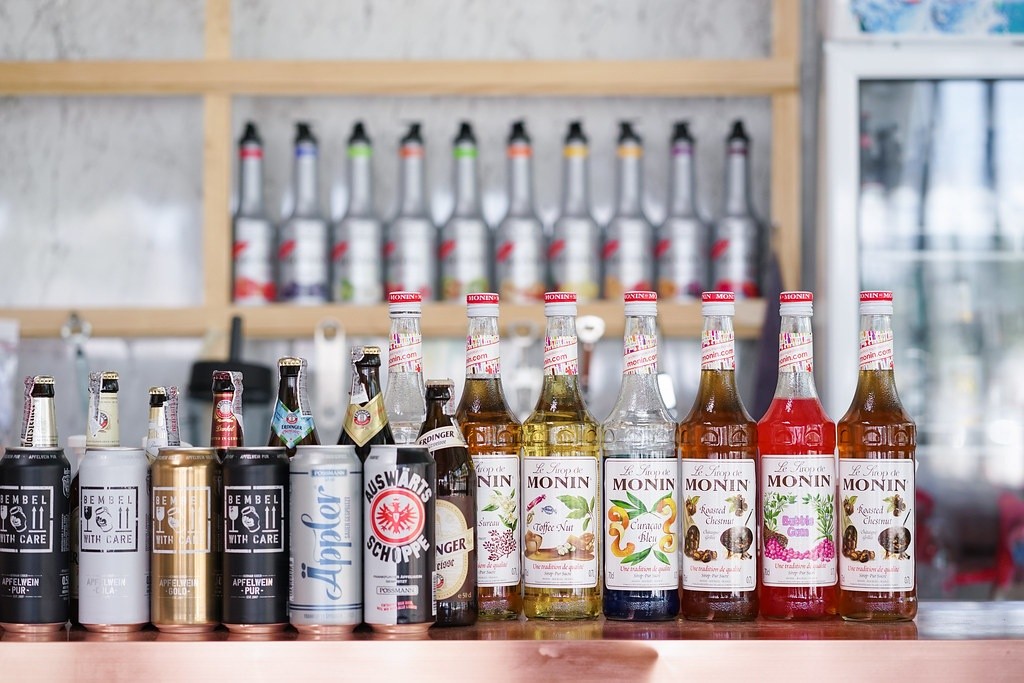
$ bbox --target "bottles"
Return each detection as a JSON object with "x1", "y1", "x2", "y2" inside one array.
[
  {"x1": 758, "y1": 292, "x2": 840, "y2": 624},
  {"x1": 231, "y1": 121, "x2": 279, "y2": 310},
  {"x1": 338, "y1": 346, "x2": 396, "y2": 462},
  {"x1": 211, "y1": 370, "x2": 243, "y2": 450},
  {"x1": 655, "y1": 120, "x2": 709, "y2": 301},
  {"x1": 522, "y1": 293, "x2": 602, "y2": 620},
  {"x1": 272, "y1": 123, "x2": 330, "y2": 306},
  {"x1": 382, "y1": 123, "x2": 440, "y2": 304},
  {"x1": 433, "y1": 123, "x2": 494, "y2": 301},
  {"x1": 710, "y1": 117, "x2": 762, "y2": 301},
  {"x1": 414, "y1": 379, "x2": 477, "y2": 628},
  {"x1": 547, "y1": 121, "x2": 604, "y2": 302},
  {"x1": 599, "y1": 291, "x2": 680, "y2": 623},
  {"x1": 268, "y1": 358, "x2": 321, "y2": 456},
  {"x1": 19, "y1": 376, "x2": 59, "y2": 448},
  {"x1": 383, "y1": 291, "x2": 429, "y2": 445},
  {"x1": 602, "y1": 122, "x2": 655, "y2": 304},
  {"x1": 491, "y1": 121, "x2": 550, "y2": 302},
  {"x1": 68, "y1": 372, "x2": 121, "y2": 629},
  {"x1": 454, "y1": 292, "x2": 524, "y2": 622},
  {"x1": 145, "y1": 386, "x2": 181, "y2": 463},
  {"x1": 679, "y1": 292, "x2": 760, "y2": 622},
  {"x1": 839, "y1": 291, "x2": 917, "y2": 623},
  {"x1": 331, "y1": 123, "x2": 386, "y2": 304}
]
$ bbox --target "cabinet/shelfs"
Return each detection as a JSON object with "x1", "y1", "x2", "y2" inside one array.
[{"x1": 0, "y1": 1, "x2": 803, "y2": 363}]
